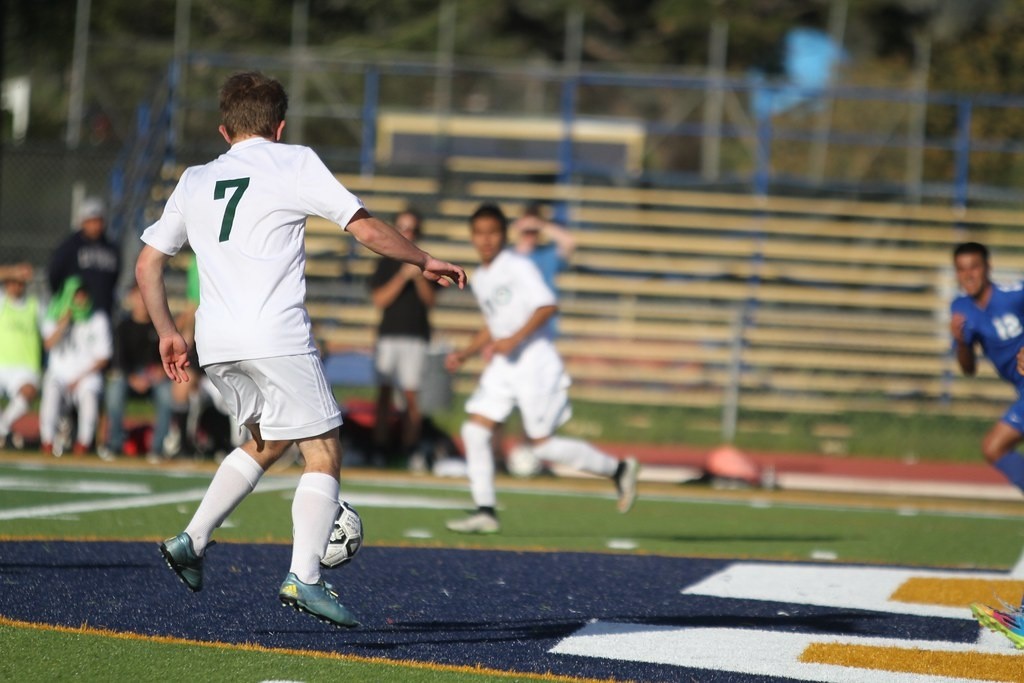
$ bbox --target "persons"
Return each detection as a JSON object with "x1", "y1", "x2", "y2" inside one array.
[
  {"x1": 0, "y1": 261, "x2": 39, "y2": 448},
  {"x1": 447, "y1": 202, "x2": 639, "y2": 535},
  {"x1": 363, "y1": 212, "x2": 432, "y2": 471},
  {"x1": 96, "y1": 255, "x2": 216, "y2": 461},
  {"x1": 950, "y1": 243, "x2": 1024, "y2": 490},
  {"x1": 40, "y1": 200, "x2": 123, "y2": 455},
  {"x1": 134, "y1": 70, "x2": 466, "y2": 627},
  {"x1": 513, "y1": 209, "x2": 576, "y2": 342},
  {"x1": 970, "y1": 599, "x2": 1024, "y2": 649}
]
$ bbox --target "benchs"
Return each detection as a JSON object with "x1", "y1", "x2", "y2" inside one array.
[{"x1": 125, "y1": 160, "x2": 1023, "y2": 421}]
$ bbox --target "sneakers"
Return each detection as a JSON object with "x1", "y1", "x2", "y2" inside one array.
[
  {"x1": 278, "y1": 572, "x2": 365, "y2": 632},
  {"x1": 445, "y1": 512, "x2": 501, "y2": 534},
  {"x1": 971, "y1": 598, "x2": 1024, "y2": 648},
  {"x1": 617, "y1": 457, "x2": 638, "y2": 515},
  {"x1": 158, "y1": 531, "x2": 216, "y2": 592}
]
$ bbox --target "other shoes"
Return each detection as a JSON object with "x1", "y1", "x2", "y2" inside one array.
[
  {"x1": 0, "y1": 432, "x2": 8, "y2": 447},
  {"x1": 39, "y1": 442, "x2": 56, "y2": 460},
  {"x1": 72, "y1": 442, "x2": 88, "y2": 457},
  {"x1": 149, "y1": 452, "x2": 161, "y2": 464},
  {"x1": 96, "y1": 443, "x2": 116, "y2": 463},
  {"x1": 11, "y1": 429, "x2": 24, "y2": 449}
]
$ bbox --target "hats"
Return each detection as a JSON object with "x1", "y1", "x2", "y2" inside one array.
[{"x1": 76, "y1": 198, "x2": 105, "y2": 220}]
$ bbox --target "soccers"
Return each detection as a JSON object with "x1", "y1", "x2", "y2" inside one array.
[{"x1": 292, "y1": 497, "x2": 364, "y2": 570}]
